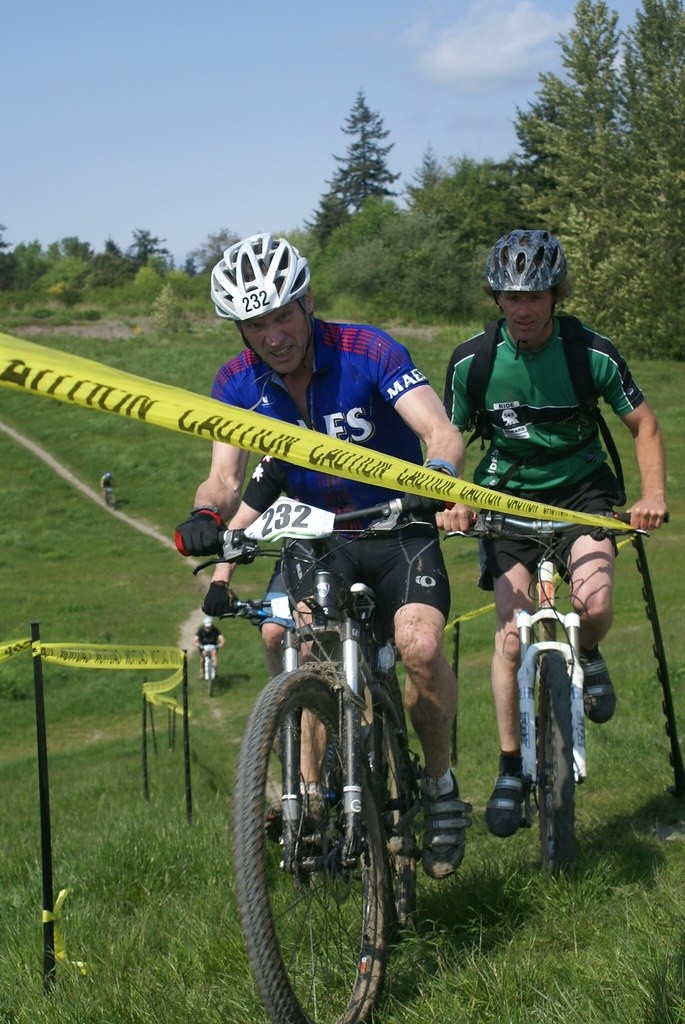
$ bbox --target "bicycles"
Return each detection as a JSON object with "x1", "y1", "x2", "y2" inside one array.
[
  {"x1": 467, "y1": 509, "x2": 672, "y2": 879},
  {"x1": 197, "y1": 644, "x2": 219, "y2": 697},
  {"x1": 102, "y1": 485, "x2": 117, "y2": 511},
  {"x1": 192, "y1": 511, "x2": 505, "y2": 1023}
]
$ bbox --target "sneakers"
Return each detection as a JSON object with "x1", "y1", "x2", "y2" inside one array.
[
  {"x1": 485, "y1": 760, "x2": 526, "y2": 838},
  {"x1": 421, "y1": 770, "x2": 473, "y2": 880},
  {"x1": 264, "y1": 792, "x2": 327, "y2": 848},
  {"x1": 579, "y1": 649, "x2": 616, "y2": 724}
]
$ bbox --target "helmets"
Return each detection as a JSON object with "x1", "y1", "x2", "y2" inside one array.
[
  {"x1": 210, "y1": 232, "x2": 311, "y2": 321},
  {"x1": 487, "y1": 229, "x2": 567, "y2": 291},
  {"x1": 202, "y1": 617, "x2": 213, "y2": 628},
  {"x1": 104, "y1": 473, "x2": 112, "y2": 480}
]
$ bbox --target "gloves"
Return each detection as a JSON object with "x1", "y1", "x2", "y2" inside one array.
[
  {"x1": 406, "y1": 464, "x2": 455, "y2": 518},
  {"x1": 174, "y1": 505, "x2": 225, "y2": 556},
  {"x1": 200, "y1": 580, "x2": 231, "y2": 617}
]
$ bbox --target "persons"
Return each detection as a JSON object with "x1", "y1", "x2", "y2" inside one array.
[
  {"x1": 176, "y1": 233, "x2": 465, "y2": 881},
  {"x1": 435, "y1": 229, "x2": 668, "y2": 838},
  {"x1": 193, "y1": 617, "x2": 225, "y2": 679},
  {"x1": 101, "y1": 473, "x2": 114, "y2": 499}
]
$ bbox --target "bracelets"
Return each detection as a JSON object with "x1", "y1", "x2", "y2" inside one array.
[{"x1": 425, "y1": 459, "x2": 457, "y2": 477}]
